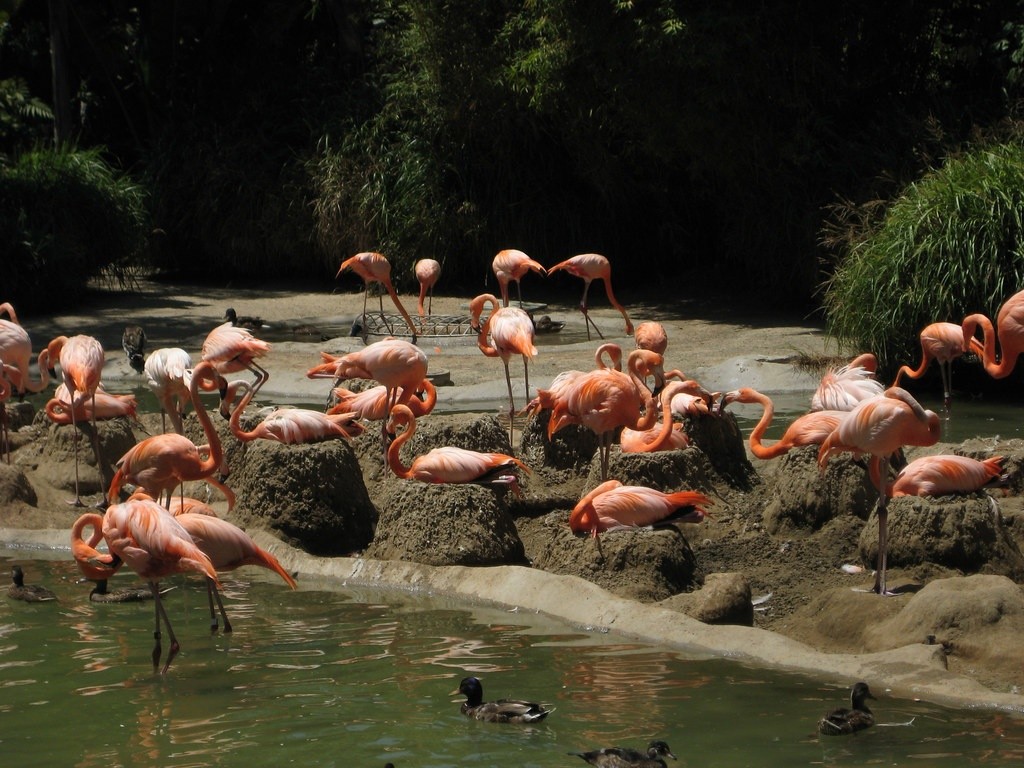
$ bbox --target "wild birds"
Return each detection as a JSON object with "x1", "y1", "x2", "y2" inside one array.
[
  {"x1": 89, "y1": 579, "x2": 179, "y2": 603},
  {"x1": 106, "y1": 363, "x2": 228, "y2": 510},
  {"x1": 122, "y1": 325, "x2": 146, "y2": 361},
  {"x1": 0, "y1": 302, "x2": 437, "y2": 465},
  {"x1": 809, "y1": 290, "x2": 1024, "y2": 411},
  {"x1": 70, "y1": 489, "x2": 297, "y2": 651},
  {"x1": 468, "y1": 254, "x2": 722, "y2": 484},
  {"x1": 222, "y1": 307, "x2": 264, "y2": 330},
  {"x1": 8, "y1": 565, "x2": 59, "y2": 603},
  {"x1": 383, "y1": 403, "x2": 532, "y2": 486},
  {"x1": 568, "y1": 741, "x2": 677, "y2": 768},
  {"x1": 334, "y1": 252, "x2": 417, "y2": 346},
  {"x1": 869, "y1": 453, "x2": 1004, "y2": 498},
  {"x1": 569, "y1": 480, "x2": 716, "y2": 538},
  {"x1": 493, "y1": 250, "x2": 547, "y2": 309},
  {"x1": 818, "y1": 386, "x2": 941, "y2": 596},
  {"x1": 818, "y1": 682, "x2": 878, "y2": 736},
  {"x1": 416, "y1": 259, "x2": 441, "y2": 323},
  {"x1": 718, "y1": 388, "x2": 850, "y2": 460},
  {"x1": 448, "y1": 677, "x2": 556, "y2": 724}
]
